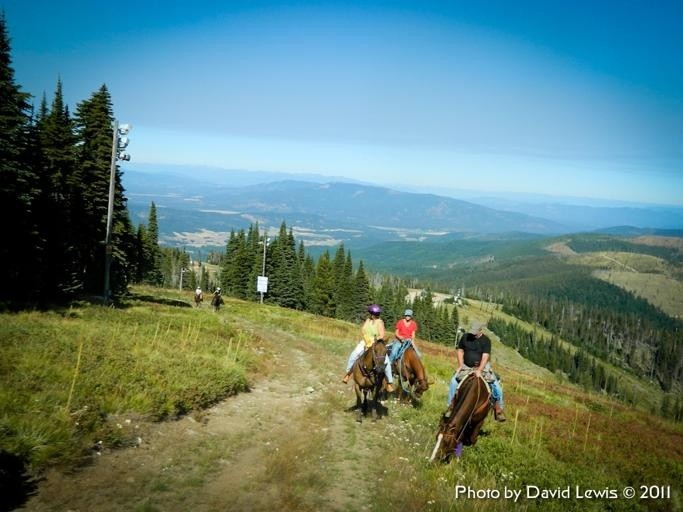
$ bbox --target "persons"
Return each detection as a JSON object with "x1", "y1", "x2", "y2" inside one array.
[
  {"x1": 195, "y1": 286, "x2": 202, "y2": 296},
  {"x1": 442, "y1": 319, "x2": 507, "y2": 422},
  {"x1": 385, "y1": 307, "x2": 436, "y2": 385},
  {"x1": 341, "y1": 304, "x2": 395, "y2": 393},
  {"x1": 210, "y1": 286, "x2": 225, "y2": 306}
]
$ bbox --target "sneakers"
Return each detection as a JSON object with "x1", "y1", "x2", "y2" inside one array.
[
  {"x1": 341, "y1": 374, "x2": 350, "y2": 384},
  {"x1": 444, "y1": 407, "x2": 451, "y2": 418},
  {"x1": 495, "y1": 408, "x2": 506, "y2": 422},
  {"x1": 385, "y1": 384, "x2": 394, "y2": 393},
  {"x1": 426, "y1": 379, "x2": 434, "y2": 385}
]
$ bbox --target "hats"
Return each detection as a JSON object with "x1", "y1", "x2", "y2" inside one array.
[
  {"x1": 404, "y1": 309, "x2": 413, "y2": 316},
  {"x1": 466, "y1": 320, "x2": 480, "y2": 336}
]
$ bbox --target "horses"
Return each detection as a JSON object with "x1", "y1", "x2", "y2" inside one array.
[
  {"x1": 194, "y1": 293, "x2": 203, "y2": 307},
  {"x1": 387, "y1": 341, "x2": 434, "y2": 404},
  {"x1": 428, "y1": 372, "x2": 493, "y2": 465},
  {"x1": 352, "y1": 334, "x2": 392, "y2": 426},
  {"x1": 212, "y1": 291, "x2": 222, "y2": 312}
]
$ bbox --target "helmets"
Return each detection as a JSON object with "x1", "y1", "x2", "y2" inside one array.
[{"x1": 367, "y1": 303, "x2": 381, "y2": 313}]
